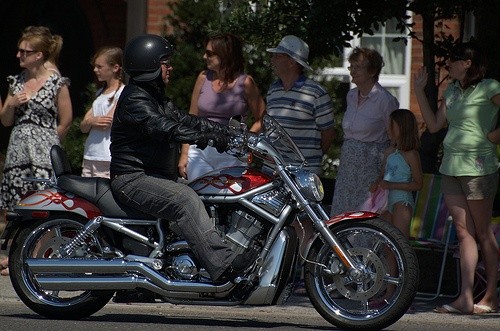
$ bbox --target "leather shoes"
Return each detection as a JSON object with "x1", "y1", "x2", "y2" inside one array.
[{"x1": 217, "y1": 243, "x2": 261, "y2": 286}]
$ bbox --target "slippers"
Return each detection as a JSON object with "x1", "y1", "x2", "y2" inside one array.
[
  {"x1": 433, "y1": 305, "x2": 466, "y2": 314},
  {"x1": 472, "y1": 303, "x2": 495, "y2": 314}
]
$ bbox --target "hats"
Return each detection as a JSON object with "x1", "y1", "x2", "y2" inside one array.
[{"x1": 266, "y1": 35, "x2": 314, "y2": 71}]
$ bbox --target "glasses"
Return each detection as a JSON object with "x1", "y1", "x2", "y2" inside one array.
[
  {"x1": 206, "y1": 49, "x2": 217, "y2": 57},
  {"x1": 449, "y1": 56, "x2": 467, "y2": 63},
  {"x1": 160, "y1": 59, "x2": 170, "y2": 68},
  {"x1": 20, "y1": 49, "x2": 37, "y2": 55},
  {"x1": 348, "y1": 65, "x2": 366, "y2": 71}
]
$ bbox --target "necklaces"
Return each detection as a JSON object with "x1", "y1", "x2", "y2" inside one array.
[{"x1": 359, "y1": 91, "x2": 367, "y2": 99}]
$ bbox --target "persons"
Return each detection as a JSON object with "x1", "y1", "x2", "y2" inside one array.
[
  {"x1": 260, "y1": 34, "x2": 337, "y2": 297},
  {"x1": 363, "y1": 109, "x2": 426, "y2": 308},
  {"x1": 415, "y1": 39, "x2": 500, "y2": 319},
  {"x1": 78, "y1": 47, "x2": 129, "y2": 183},
  {"x1": 0, "y1": 24, "x2": 74, "y2": 275},
  {"x1": 108, "y1": 35, "x2": 268, "y2": 284},
  {"x1": 173, "y1": 33, "x2": 267, "y2": 222},
  {"x1": 323, "y1": 47, "x2": 400, "y2": 246}
]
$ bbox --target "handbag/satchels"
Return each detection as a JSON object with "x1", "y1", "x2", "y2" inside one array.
[{"x1": 362, "y1": 185, "x2": 388, "y2": 213}]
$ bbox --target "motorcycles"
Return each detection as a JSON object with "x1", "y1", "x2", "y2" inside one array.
[{"x1": 0, "y1": 111, "x2": 420, "y2": 331}]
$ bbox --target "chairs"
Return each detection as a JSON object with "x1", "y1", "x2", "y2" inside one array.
[
  {"x1": 455, "y1": 216, "x2": 500, "y2": 306},
  {"x1": 406, "y1": 174, "x2": 462, "y2": 301}
]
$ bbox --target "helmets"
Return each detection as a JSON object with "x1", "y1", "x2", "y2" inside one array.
[{"x1": 123, "y1": 35, "x2": 172, "y2": 72}]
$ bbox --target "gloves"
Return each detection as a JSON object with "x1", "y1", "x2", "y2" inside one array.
[
  {"x1": 196, "y1": 133, "x2": 227, "y2": 154},
  {"x1": 228, "y1": 128, "x2": 257, "y2": 138}
]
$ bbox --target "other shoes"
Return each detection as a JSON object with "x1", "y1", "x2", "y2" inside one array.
[{"x1": 0, "y1": 268, "x2": 9, "y2": 275}]
